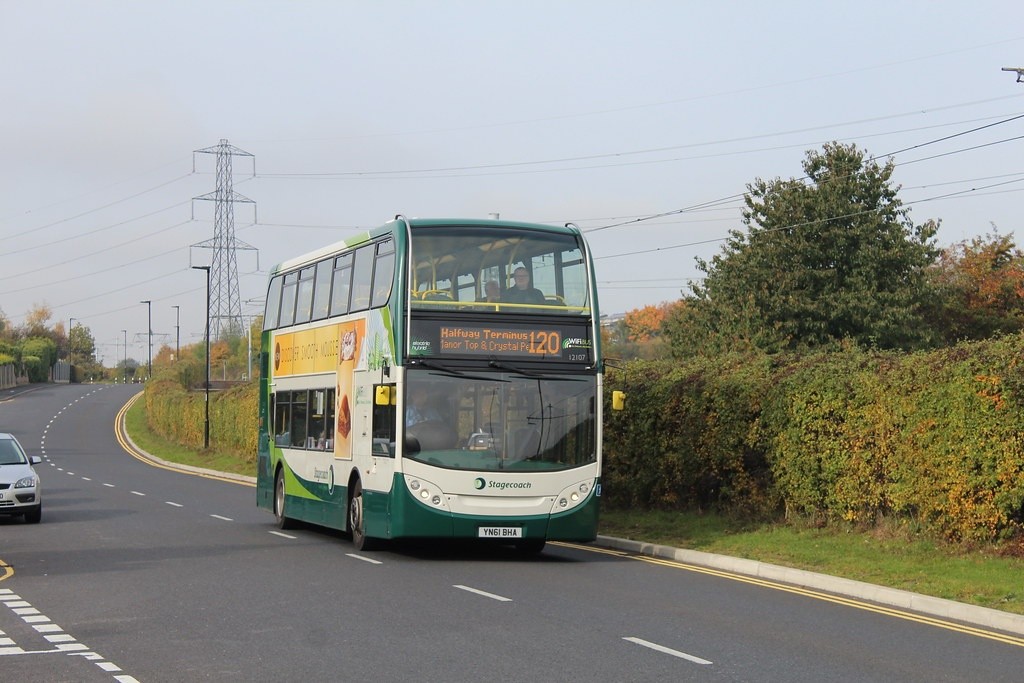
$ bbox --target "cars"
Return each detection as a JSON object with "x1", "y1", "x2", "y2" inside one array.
[{"x1": 0, "y1": 432, "x2": 43, "y2": 523}]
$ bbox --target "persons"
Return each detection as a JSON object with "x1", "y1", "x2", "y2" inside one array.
[
  {"x1": 404, "y1": 386, "x2": 441, "y2": 429},
  {"x1": 482, "y1": 267, "x2": 544, "y2": 305}
]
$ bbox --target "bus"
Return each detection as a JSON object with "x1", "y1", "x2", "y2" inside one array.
[{"x1": 255, "y1": 213, "x2": 629, "y2": 557}]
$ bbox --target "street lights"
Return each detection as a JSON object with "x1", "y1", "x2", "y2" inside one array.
[
  {"x1": 139, "y1": 301, "x2": 151, "y2": 377},
  {"x1": 120, "y1": 329, "x2": 127, "y2": 384},
  {"x1": 192, "y1": 266, "x2": 211, "y2": 449},
  {"x1": 69, "y1": 317, "x2": 77, "y2": 365},
  {"x1": 171, "y1": 305, "x2": 180, "y2": 360}
]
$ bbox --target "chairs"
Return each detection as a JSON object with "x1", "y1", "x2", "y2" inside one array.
[
  {"x1": 273, "y1": 421, "x2": 335, "y2": 451},
  {"x1": 270, "y1": 289, "x2": 568, "y2": 328}
]
